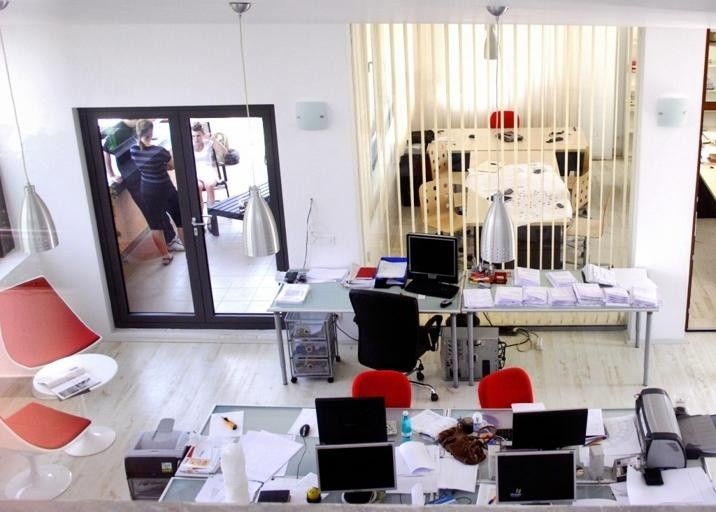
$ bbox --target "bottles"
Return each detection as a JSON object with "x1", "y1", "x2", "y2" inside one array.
[{"x1": 400, "y1": 410, "x2": 412, "y2": 439}]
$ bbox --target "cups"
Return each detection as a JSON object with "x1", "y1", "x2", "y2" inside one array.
[{"x1": 237, "y1": 198, "x2": 247, "y2": 213}]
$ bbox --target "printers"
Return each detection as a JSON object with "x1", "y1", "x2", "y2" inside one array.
[
  {"x1": 635, "y1": 387, "x2": 715, "y2": 468},
  {"x1": 123, "y1": 417, "x2": 193, "y2": 500}
]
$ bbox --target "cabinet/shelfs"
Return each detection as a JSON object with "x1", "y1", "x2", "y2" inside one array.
[
  {"x1": 622, "y1": 26, "x2": 637, "y2": 157},
  {"x1": 466, "y1": 150, "x2": 570, "y2": 269},
  {"x1": 284, "y1": 312, "x2": 340, "y2": 383}
]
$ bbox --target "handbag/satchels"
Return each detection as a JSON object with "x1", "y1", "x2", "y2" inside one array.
[{"x1": 439, "y1": 427, "x2": 486, "y2": 464}]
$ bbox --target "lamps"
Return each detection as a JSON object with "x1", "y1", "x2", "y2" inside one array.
[
  {"x1": 0, "y1": 0, "x2": 59, "y2": 254},
  {"x1": 229, "y1": 2, "x2": 282, "y2": 258},
  {"x1": 480, "y1": 5, "x2": 516, "y2": 263},
  {"x1": 484, "y1": 24, "x2": 498, "y2": 60}
]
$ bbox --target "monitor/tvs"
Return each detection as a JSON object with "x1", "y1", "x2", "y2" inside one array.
[
  {"x1": 314, "y1": 397, "x2": 388, "y2": 445},
  {"x1": 494, "y1": 449, "x2": 576, "y2": 505},
  {"x1": 314, "y1": 443, "x2": 396, "y2": 503},
  {"x1": 512, "y1": 409, "x2": 589, "y2": 448},
  {"x1": 404, "y1": 234, "x2": 459, "y2": 298}
]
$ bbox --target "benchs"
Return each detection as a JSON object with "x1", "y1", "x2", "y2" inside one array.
[{"x1": 208, "y1": 183, "x2": 270, "y2": 236}]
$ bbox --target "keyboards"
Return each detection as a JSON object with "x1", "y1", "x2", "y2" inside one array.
[
  {"x1": 493, "y1": 428, "x2": 513, "y2": 447},
  {"x1": 386, "y1": 419, "x2": 398, "y2": 436},
  {"x1": 405, "y1": 278, "x2": 460, "y2": 299}
]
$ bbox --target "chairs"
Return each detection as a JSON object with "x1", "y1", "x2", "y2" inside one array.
[
  {"x1": 490, "y1": 111, "x2": 519, "y2": 128},
  {"x1": 190, "y1": 122, "x2": 230, "y2": 204},
  {"x1": 349, "y1": 289, "x2": 443, "y2": 401},
  {"x1": 352, "y1": 370, "x2": 411, "y2": 408},
  {"x1": 478, "y1": 367, "x2": 533, "y2": 408},
  {"x1": 565, "y1": 190, "x2": 614, "y2": 269},
  {"x1": 564, "y1": 169, "x2": 589, "y2": 249},
  {"x1": 0, "y1": 402, "x2": 92, "y2": 501},
  {"x1": 418, "y1": 178, "x2": 473, "y2": 269},
  {"x1": 429, "y1": 141, "x2": 469, "y2": 215},
  {"x1": 0, "y1": 275, "x2": 103, "y2": 370}
]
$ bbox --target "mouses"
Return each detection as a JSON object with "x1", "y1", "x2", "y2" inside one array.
[
  {"x1": 300, "y1": 424, "x2": 310, "y2": 438},
  {"x1": 472, "y1": 412, "x2": 483, "y2": 425},
  {"x1": 440, "y1": 298, "x2": 452, "y2": 308}
]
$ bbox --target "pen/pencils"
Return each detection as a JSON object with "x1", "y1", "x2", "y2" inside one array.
[{"x1": 488, "y1": 496, "x2": 497, "y2": 504}]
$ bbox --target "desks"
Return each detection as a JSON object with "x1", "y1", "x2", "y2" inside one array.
[
  {"x1": 33, "y1": 353, "x2": 118, "y2": 457},
  {"x1": 404, "y1": 127, "x2": 589, "y2": 206},
  {"x1": 461, "y1": 269, "x2": 660, "y2": 386},
  {"x1": 266, "y1": 268, "x2": 466, "y2": 386},
  {"x1": 700, "y1": 131, "x2": 716, "y2": 201},
  {"x1": 434, "y1": 408, "x2": 716, "y2": 505},
  {"x1": 158, "y1": 406, "x2": 447, "y2": 505}
]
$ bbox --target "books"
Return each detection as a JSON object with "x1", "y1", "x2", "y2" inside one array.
[{"x1": 356, "y1": 266, "x2": 377, "y2": 280}]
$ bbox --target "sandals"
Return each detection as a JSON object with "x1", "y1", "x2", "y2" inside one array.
[{"x1": 162, "y1": 255, "x2": 173, "y2": 265}]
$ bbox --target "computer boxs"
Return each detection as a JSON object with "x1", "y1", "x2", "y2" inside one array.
[{"x1": 442, "y1": 326, "x2": 498, "y2": 381}]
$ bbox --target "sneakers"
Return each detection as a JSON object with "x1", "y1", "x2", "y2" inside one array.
[{"x1": 167, "y1": 236, "x2": 185, "y2": 251}]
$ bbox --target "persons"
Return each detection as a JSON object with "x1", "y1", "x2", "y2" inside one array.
[
  {"x1": 129, "y1": 118, "x2": 185, "y2": 265},
  {"x1": 109, "y1": 119, "x2": 186, "y2": 252},
  {"x1": 191, "y1": 121, "x2": 227, "y2": 217}
]
